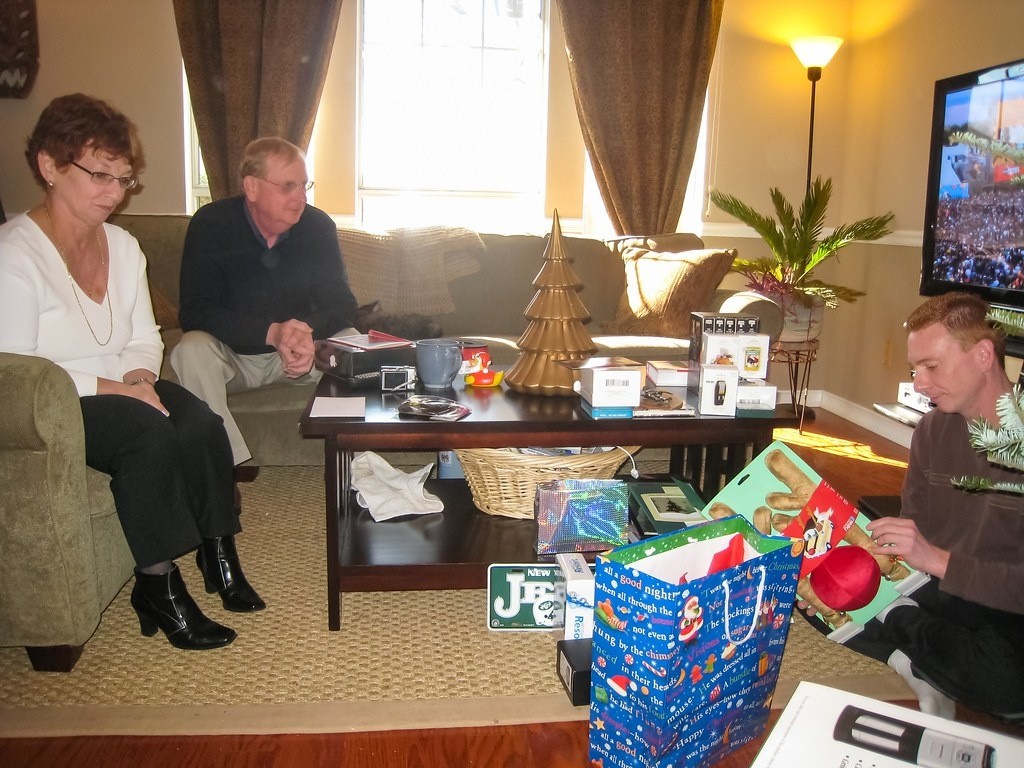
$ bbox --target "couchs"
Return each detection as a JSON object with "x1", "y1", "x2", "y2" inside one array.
[{"x1": 0, "y1": 214, "x2": 783, "y2": 671}]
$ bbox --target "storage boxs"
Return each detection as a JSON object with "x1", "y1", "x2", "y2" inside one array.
[
  {"x1": 580, "y1": 399, "x2": 633, "y2": 419},
  {"x1": 689, "y1": 312, "x2": 777, "y2": 418},
  {"x1": 749, "y1": 680, "x2": 1024, "y2": 768},
  {"x1": 555, "y1": 553, "x2": 595, "y2": 640},
  {"x1": 557, "y1": 638, "x2": 593, "y2": 707},
  {"x1": 580, "y1": 368, "x2": 641, "y2": 407}
]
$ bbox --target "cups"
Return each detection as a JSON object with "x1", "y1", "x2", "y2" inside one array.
[{"x1": 416, "y1": 338, "x2": 463, "y2": 389}]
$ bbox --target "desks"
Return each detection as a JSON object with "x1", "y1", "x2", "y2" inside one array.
[{"x1": 771, "y1": 339, "x2": 820, "y2": 435}]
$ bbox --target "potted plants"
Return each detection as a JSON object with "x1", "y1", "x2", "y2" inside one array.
[{"x1": 711, "y1": 175, "x2": 895, "y2": 342}]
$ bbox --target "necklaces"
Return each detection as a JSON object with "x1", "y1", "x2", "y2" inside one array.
[{"x1": 47, "y1": 209, "x2": 113, "y2": 346}]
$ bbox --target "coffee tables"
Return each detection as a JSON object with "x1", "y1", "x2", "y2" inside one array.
[{"x1": 297, "y1": 361, "x2": 800, "y2": 631}]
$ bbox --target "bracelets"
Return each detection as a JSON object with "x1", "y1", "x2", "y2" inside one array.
[{"x1": 129, "y1": 376, "x2": 153, "y2": 386}]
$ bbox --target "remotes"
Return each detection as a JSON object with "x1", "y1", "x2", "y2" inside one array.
[{"x1": 349, "y1": 372, "x2": 382, "y2": 388}]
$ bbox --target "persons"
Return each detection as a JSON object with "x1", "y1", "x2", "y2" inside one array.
[
  {"x1": 170, "y1": 135, "x2": 363, "y2": 473},
  {"x1": 0, "y1": 93, "x2": 268, "y2": 649},
  {"x1": 929, "y1": 188, "x2": 1024, "y2": 290},
  {"x1": 797, "y1": 290, "x2": 1022, "y2": 724}
]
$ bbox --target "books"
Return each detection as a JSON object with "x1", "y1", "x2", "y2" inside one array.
[
  {"x1": 702, "y1": 441, "x2": 927, "y2": 639},
  {"x1": 632, "y1": 390, "x2": 696, "y2": 416},
  {"x1": 624, "y1": 477, "x2": 711, "y2": 537}
]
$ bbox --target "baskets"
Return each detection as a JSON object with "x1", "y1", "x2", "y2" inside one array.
[{"x1": 453, "y1": 446, "x2": 641, "y2": 520}]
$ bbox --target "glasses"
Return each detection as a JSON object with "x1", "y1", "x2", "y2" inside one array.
[
  {"x1": 255, "y1": 175, "x2": 314, "y2": 193},
  {"x1": 70, "y1": 160, "x2": 139, "y2": 189}
]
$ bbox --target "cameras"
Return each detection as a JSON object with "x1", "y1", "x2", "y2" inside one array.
[{"x1": 380, "y1": 365, "x2": 418, "y2": 391}]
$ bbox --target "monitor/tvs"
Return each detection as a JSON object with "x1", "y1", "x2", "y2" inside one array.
[{"x1": 918, "y1": 58, "x2": 1024, "y2": 342}]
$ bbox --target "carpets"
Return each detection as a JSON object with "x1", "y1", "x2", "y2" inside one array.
[{"x1": 0, "y1": 463, "x2": 919, "y2": 737}]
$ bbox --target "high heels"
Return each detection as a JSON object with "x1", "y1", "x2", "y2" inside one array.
[
  {"x1": 130, "y1": 562, "x2": 238, "y2": 650},
  {"x1": 196, "y1": 534, "x2": 266, "y2": 612}
]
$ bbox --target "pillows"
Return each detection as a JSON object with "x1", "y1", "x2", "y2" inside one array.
[{"x1": 600, "y1": 246, "x2": 737, "y2": 339}]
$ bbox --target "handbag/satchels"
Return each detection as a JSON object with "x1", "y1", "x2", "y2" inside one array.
[
  {"x1": 588, "y1": 515, "x2": 806, "y2": 768},
  {"x1": 532, "y1": 478, "x2": 629, "y2": 555}
]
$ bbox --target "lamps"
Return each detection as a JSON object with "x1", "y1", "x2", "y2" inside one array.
[{"x1": 777, "y1": 36, "x2": 844, "y2": 421}]
